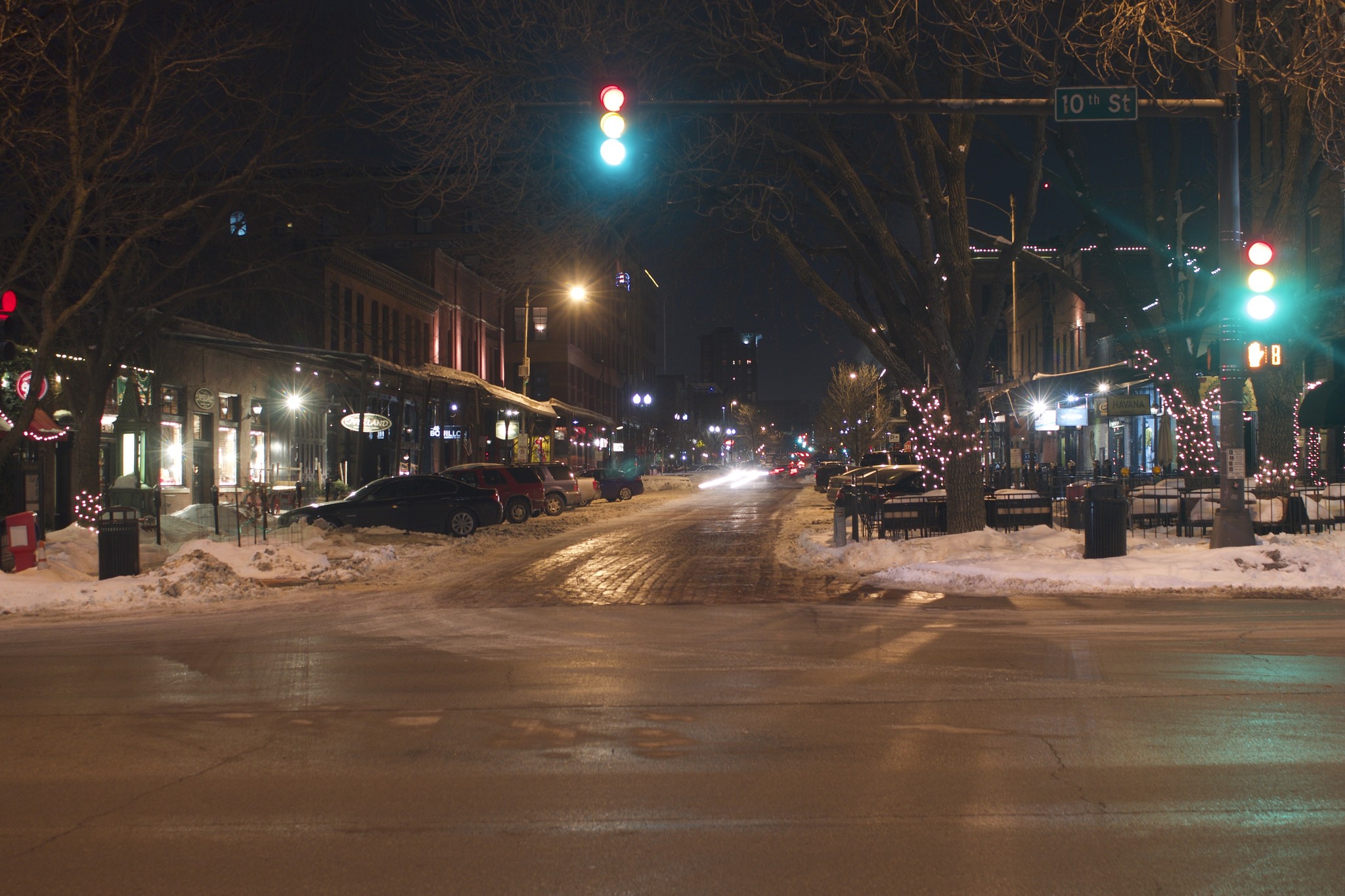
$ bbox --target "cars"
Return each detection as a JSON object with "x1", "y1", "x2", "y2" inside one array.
[
  {"x1": 277, "y1": 474, "x2": 503, "y2": 538},
  {"x1": 649, "y1": 452, "x2": 812, "y2": 476},
  {"x1": 835, "y1": 464, "x2": 998, "y2": 517},
  {"x1": 574, "y1": 474, "x2": 601, "y2": 507},
  {"x1": 574, "y1": 468, "x2": 644, "y2": 502},
  {"x1": 827, "y1": 465, "x2": 895, "y2": 504}
]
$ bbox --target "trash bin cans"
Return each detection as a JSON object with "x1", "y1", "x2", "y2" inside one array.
[
  {"x1": 6, "y1": 511, "x2": 38, "y2": 573},
  {"x1": 1082, "y1": 483, "x2": 1129, "y2": 559},
  {"x1": 98, "y1": 506, "x2": 140, "y2": 582}
]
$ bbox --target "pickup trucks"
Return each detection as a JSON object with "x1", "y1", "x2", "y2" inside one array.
[{"x1": 814, "y1": 451, "x2": 921, "y2": 493}]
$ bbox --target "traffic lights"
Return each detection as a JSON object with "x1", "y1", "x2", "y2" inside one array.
[
  {"x1": 1241, "y1": 240, "x2": 1285, "y2": 372},
  {"x1": 592, "y1": 77, "x2": 638, "y2": 185}
]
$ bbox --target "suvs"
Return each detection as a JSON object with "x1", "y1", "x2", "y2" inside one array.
[
  {"x1": 522, "y1": 461, "x2": 582, "y2": 517},
  {"x1": 438, "y1": 463, "x2": 544, "y2": 523}
]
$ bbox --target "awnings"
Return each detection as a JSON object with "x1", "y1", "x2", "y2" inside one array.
[
  {"x1": 1298, "y1": 379, "x2": 1345, "y2": 430},
  {"x1": 1, "y1": 408, "x2": 68, "y2": 441},
  {"x1": 979, "y1": 358, "x2": 1152, "y2": 495},
  {"x1": 167, "y1": 332, "x2": 612, "y2": 499}
]
